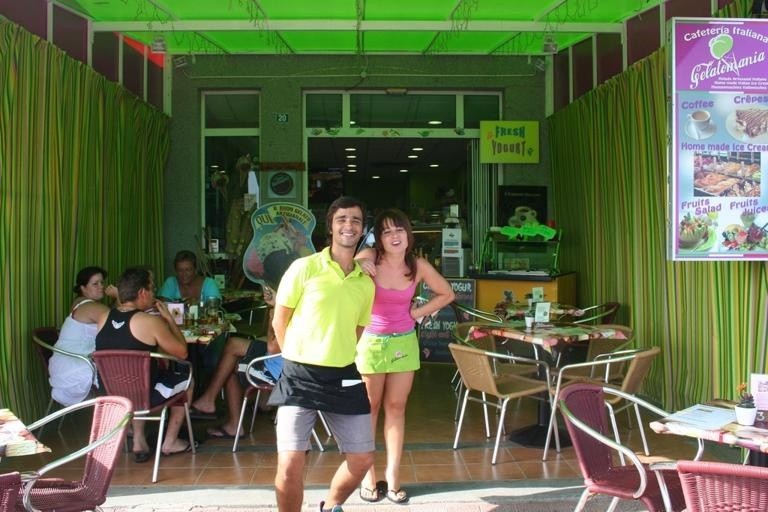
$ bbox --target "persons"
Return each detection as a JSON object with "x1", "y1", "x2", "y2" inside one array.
[
  {"x1": 192, "y1": 287, "x2": 284, "y2": 440},
  {"x1": 266, "y1": 196, "x2": 376, "y2": 512},
  {"x1": 354, "y1": 208, "x2": 455, "y2": 504},
  {"x1": 95, "y1": 267, "x2": 199, "y2": 463},
  {"x1": 157, "y1": 250, "x2": 223, "y2": 385},
  {"x1": 47, "y1": 266, "x2": 120, "y2": 408}
]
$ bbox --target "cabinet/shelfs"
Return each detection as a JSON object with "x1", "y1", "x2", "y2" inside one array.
[{"x1": 472, "y1": 229, "x2": 577, "y2": 323}]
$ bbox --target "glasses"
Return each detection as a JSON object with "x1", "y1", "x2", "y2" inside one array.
[
  {"x1": 535, "y1": 58, "x2": 545, "y2": 72},
  {"x1": 543, "y1": 34, "x2": 558, "y2": 54},
  {"x1": 151, "y1": 36, "x2": 167, "y2": 52},
  {"x1": 174, "y1": 55, "x2": 187, "y2": 68}
]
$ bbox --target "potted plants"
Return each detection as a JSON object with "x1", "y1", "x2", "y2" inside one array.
[{"x1": 666, "y1": 14, "x2": 767, "y2": 260}]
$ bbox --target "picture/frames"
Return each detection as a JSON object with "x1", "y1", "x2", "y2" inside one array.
[{"x1": 666, "y1": 14, "x2": 767, "y2": 260}]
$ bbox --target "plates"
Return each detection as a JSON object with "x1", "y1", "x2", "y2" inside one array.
[{"x1": 472, "y1": 229, "x2": 577, "y2": 323}]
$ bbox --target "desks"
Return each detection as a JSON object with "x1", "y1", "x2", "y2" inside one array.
[
  {"x1": 1, "y1": 407, "x2": 51, "y2": 474},
  {"x1": 649, "y1": 398, "x2": 768, "y2": 466}
]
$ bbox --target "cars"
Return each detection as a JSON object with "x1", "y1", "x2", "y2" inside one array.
[
  {"x1": 206, "y1": 310, "x2": 223, "y2": 325},
  {"x1": 184, "y1": 314, "x2": 194, "y2": 326}
]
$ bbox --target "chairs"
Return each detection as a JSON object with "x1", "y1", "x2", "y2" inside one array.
[
  {"x1": 2, "y1": 470, "x2": 21, "y2": 511},
  {"x1": 557, "y1": 384, "x2": 705, "y2": 509},
  {"x1": 676, "y1": 459, "x2": 768, "y2": 510},
  {"x1": 29, "y1": 287, "x2": 333, "y2": 484},
  {"x1": 320, "y1": 501, "x2": 343, "y2": 512},
  {"x1": 15, "y1": 396, "x2": 134, "y2": 511},
  {"x1": 449, "y1": 300, "x2": 660, "y2": 466}
]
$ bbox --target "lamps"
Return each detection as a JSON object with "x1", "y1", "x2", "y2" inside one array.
[
  {"x1": 543, "y1": 34, "x2": 558, "y2": 54},
  {"x1": 151, "y1": 36, "x2": 167, "y2": 52},
  {"x1": 535, "y1": 58, "x2": 545, "y2": 72},
  {"x1": 174, "y1": 55, "x2": 187, "y2": 68}
]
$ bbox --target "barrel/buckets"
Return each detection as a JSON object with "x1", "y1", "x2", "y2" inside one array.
[
  {"x1": 359, "y1": 485, "x2": 379, "y2": 502},
  {"x1": 189, "y1": 403, "x2": 217, "y2": 420},
  {"x1": 205, "y1": 425, "x2": 245, "y2": 439},
  {"x1": 376, "y1": 481, "x2": 408, "y2": 503},
  {"x1": 135, "y1": 445, "x2": 154, "y2": 463},
  {"x1": 161, "y1": 438, "x2": 199, "y2": 457}
]
